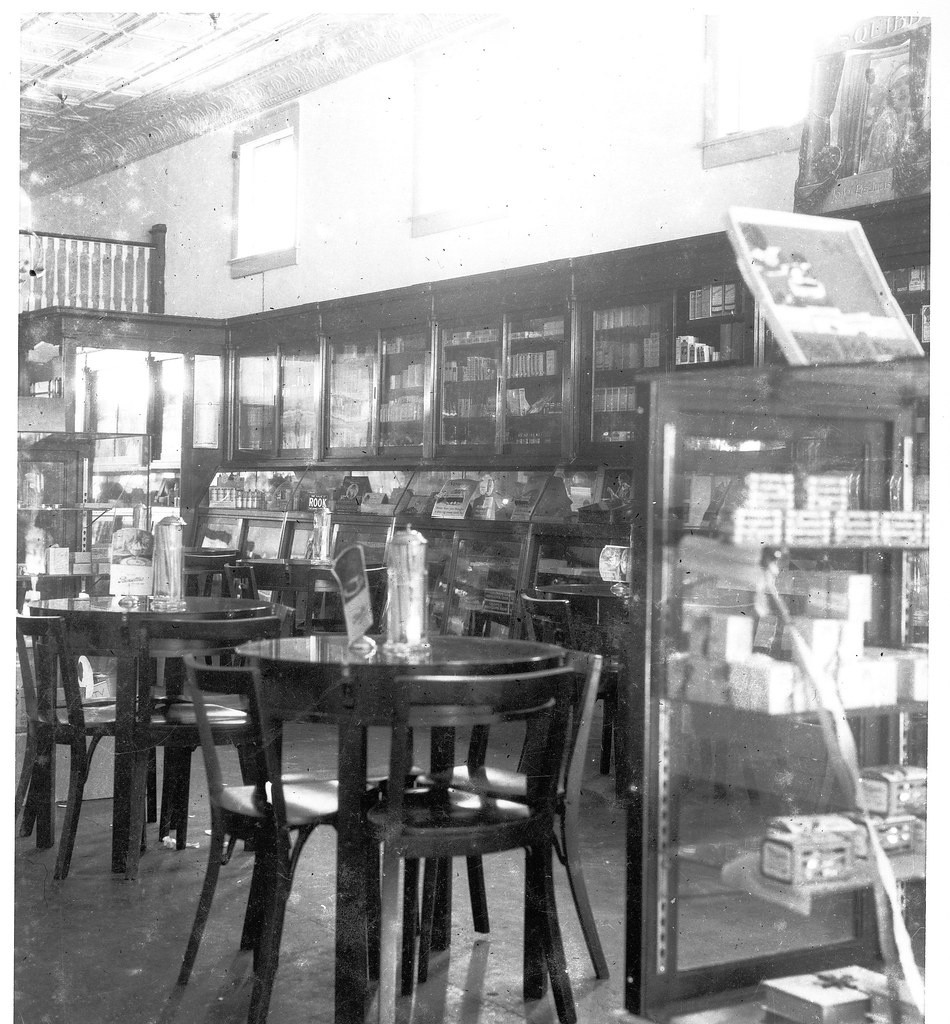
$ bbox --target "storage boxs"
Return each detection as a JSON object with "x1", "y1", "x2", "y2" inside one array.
[{"x1": 759, "y1": 760, "x2": 928, "y2": 884}]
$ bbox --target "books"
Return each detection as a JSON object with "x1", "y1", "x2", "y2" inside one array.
[{"x1": 237, "y1": 248, "x2": 931, "y2": 453}]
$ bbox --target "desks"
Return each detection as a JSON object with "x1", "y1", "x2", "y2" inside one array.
[
  {"x1": 228, "y1": 635, "x2": 568, "y2": 1023},
  {"x1": 536, "y1": 581, "x2": 630, "y2": 629},
  {"x1": 28, "y1": 593, "x2": 275, "y2": 873},
  {"x1": 235, "y1": 557, "x2": 383, "y2": 632}
]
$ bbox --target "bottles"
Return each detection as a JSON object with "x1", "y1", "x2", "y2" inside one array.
[
  {"x1": 382, "y1": 528, "x2": 432, "y2": 650},
  {"x1": 242, "y1": 491, "x2": 247, "y2": 509},
  {"x1": 236, "y1": 489, "x2": 242, "y2": 509},
  {"x1": 247, "y1": 489, "x2": 252, "y2": 509},
  {"x1": 252, "y1": 489, "x2": 258, "y2": 508},
  {"x1": 311, "y1": 508, "x2": 331, "y2": 565},
  {"x1": 149, "y1": 515, "x2": 187, "y2": 613}
]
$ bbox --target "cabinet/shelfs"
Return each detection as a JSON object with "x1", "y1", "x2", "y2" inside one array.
[
  {"x1": 625, "y1": 364, "x2": 930, "y2": 1022},
  {"x1": 226, "y1": 194, "x2": 930, "y2": 470},
  {"x1": 17, "y1": 429, "x2": 151, "y2": 580}
]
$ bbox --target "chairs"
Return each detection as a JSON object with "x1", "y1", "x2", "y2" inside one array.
[{"x1": 17, "y1": 546, "x2": 628, "y2": 1024}]
